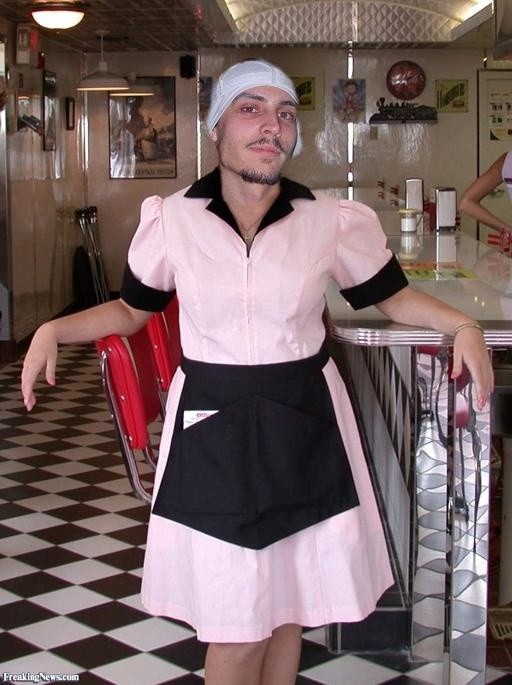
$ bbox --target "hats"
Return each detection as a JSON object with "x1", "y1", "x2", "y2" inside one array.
[{"x1": 207, "y1": 57, "x2": 298, "y2": 134}]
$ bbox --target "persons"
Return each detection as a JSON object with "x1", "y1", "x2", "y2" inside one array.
[
  {"x1": 458, "y1": 249, "x2": 512, "y2": 321},
  {"x1": 21, "y1": 60, "x2": 495, "y2": 685},
  {"x1": 460, "y1": 151, "x2": 512, "y2": 256}
]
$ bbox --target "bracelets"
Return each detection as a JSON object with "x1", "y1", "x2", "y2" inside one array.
[{"x1": 453, "y1": 322, "x2": 484, "y2": 333}]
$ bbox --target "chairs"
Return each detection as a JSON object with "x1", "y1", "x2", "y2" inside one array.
[
  {"x1": 95, "y1": 314, "x2": 166, "y2": 507},
  {"x1": 146, "y1": 293, "x2": 182, "y2": 392}
]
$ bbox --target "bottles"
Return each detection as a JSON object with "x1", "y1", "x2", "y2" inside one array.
[{"x1": 398, "y1": 208, "x2": 417, "y2": 233}]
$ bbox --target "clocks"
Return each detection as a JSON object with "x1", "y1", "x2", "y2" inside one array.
[{"x1": 387, "y1": 61, "x2": 425, "y2": 99}]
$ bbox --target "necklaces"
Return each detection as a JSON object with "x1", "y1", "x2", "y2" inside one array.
[{"x1": 238, "y1": 215, "x2": 261, "y2": 244}]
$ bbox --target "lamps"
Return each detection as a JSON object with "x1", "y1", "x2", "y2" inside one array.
[
  {"x1": 76, "y1": 30, "x2": 129, "y2": 90},
  {"x1": 32, "y1": 0, "x2": 86, "y2": 33},
  {"x1": 109, "y1": 72, "x2": 155, "y2": 96}
]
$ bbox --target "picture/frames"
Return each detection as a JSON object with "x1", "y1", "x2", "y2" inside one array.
[
  {"x1": 66, "y1": 96, "x2": 75, "y2": 129},
  {"x1": 42, "y1": 69, "x2": 56, "y2": 150},
  {"x1": 108, "y1": 75, "x2": 177, "y2": 179}
]
